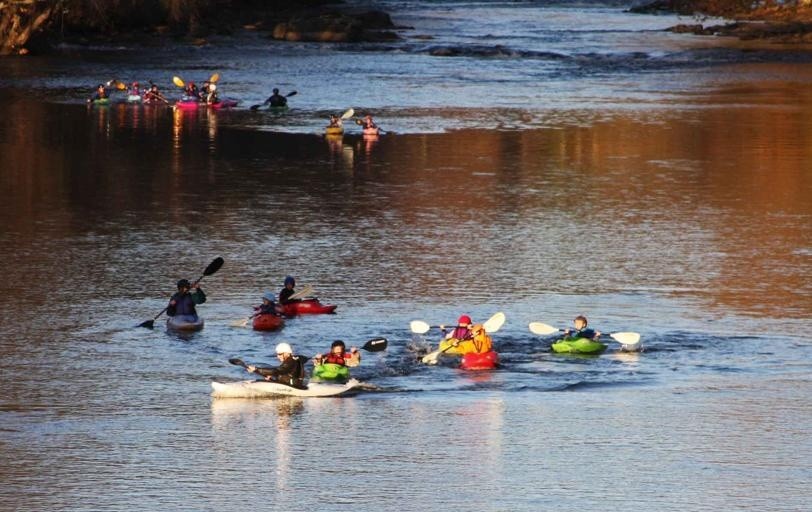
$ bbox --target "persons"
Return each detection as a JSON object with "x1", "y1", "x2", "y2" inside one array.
[
  {"x1": 440, "y1": 314, "x2": 473, "y2": 340},
  {"x1": 555, "y1": 315, "x2": 601, "y2": 343},
  {"x1": 265, "y1": 87, "x2": 288, "y2": 107},
  {"x1": 252, "y1": 292, "x2": 276, "y2": 315},
  {"x1": 314, "y1": 340, "x2": 360, "y2": 368},
  {"x1": 279, "y1": 275, "x2": 302, "y2": 304},
  {"x1": 438, "y1": 323, "x2": 491, "y2": 353},
  {"x1": 167, "y1": 280, "x2": 207, "y2": 316},
  {"x1": 247, "y1": 342, "x2": 304, "y2": 388},
  {"x1": 85, "y1": 73, "x2": 221, "y2": 108},
  {"x1": 356, "y1": 114, "x2": 377, "y2": 128},
  {"x1": 327, "y1": 115, "x2": 343, "y2": 129}
]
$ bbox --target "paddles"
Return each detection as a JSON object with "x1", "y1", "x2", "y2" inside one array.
[
  {"x1": 356, "y1": 119, "x2": 392, "y2": 134},
  {"x1": 250, "y1": 91, "x2": 298, "y2": 109},
  {"x1": 529, "y1": 322, "x2": 640, "y2": 344},
  {"x1": 292, "y1": 339, "x2": 387, "y2": 364},
  {"x1": 229, "y1": 359, "x2": 308, "y2": 390},
  {"x1": 173, "y1": 77, "x2": 198, "y2": 97},
  {"x1": 139, "y1": 257, "x2": 224, "y2": 329},
  {"x1": 209, "y1": 74, "x2": 220, "y2": 83},
  {"x1": 410, "y1": 313, "x2": 505, "y2": 365},
  {"x1": 231, "y1": 283, "x2": 312, "y2": 326},
  {"x1": 326, "y1": 109, "x2": 354, "y2": 127},
  {"x1": 115, "y1": 81, "x2": 128, "y2": 92}
]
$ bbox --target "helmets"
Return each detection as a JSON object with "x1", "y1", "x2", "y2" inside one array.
[
  {"x1": 458, "y1": 315, "x2": 471, "y2": 324},
  {"x1": 275, "y1": 343, "x2": 293, "y2": 354},
  {"x1": 284, "y1": 277, "x2": 295, "y2": 288},
  {"x1": 176, "y1": 279, "x2": 190, "y2": 290},
  {"x1": 262, "y1": 293, "x2": 276, "y2": 303}
]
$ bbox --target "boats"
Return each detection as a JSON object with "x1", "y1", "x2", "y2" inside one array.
[
  {"x1": 163, "y1": 309, "x2": 204, "y2": 336},
  {"x1": 551, "y1": 334, "x2": 609, "y2": 355},
  {"x1": 209, "y1": 376, "x2": 366, "y2": 399},
  {"x1": 325, "y1": 125, "x2": 344, "y2": 134},
  {"x1": 258, "y1": 300, "x2": 342, "y2": 319},
  {"x1": 311, "y1": 363, "x2": 351, "y2": 384},
  {"x1": 254, "y1": 311, "x2": 288, "y2": 334},
  {"x1": 363, "y1": 128, "x2": 379, "y2": 135},
  {"x1": 81, "y1": 88, "x2": 293, "y2": 112},
  {"x1": 438, "y1": 329, "x2": 493, "y2": 359}
]
